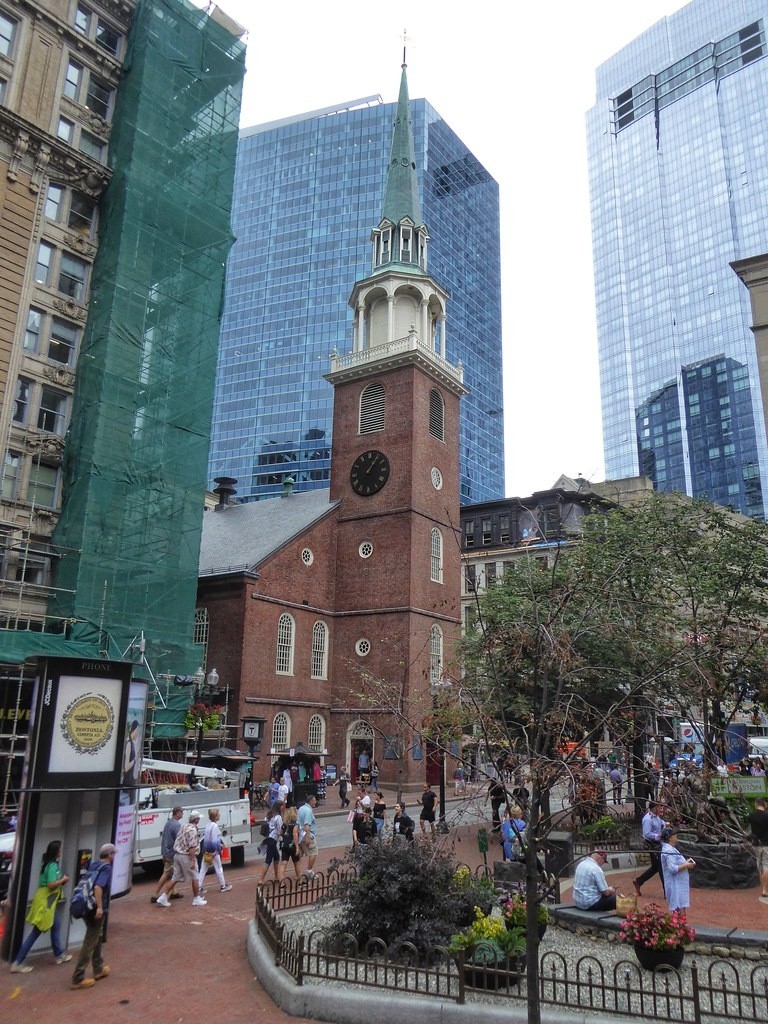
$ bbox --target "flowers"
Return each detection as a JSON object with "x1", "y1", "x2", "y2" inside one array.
[
  {"x1": 503, "y1": 894, "x2": 551, "y2": 923},
  {"x1": 623, "y1": 901, "x2": 696, "y2": 947},
  {"x1": 444, "y1": 865, "x2": 503, "y2": 902},
  {"x1": 582, "y1": 814, "x2": 624, "y2": 835},
  {"x1": 444, "y1": 914, "x2": 533, "y2": 968},
  {"x1": 184, "y1": 704, "x2": 229, "y2": 730}
]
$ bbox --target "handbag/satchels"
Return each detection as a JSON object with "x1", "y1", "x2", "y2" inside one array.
[
  {"x1": 347, "y1": 781, "x2": 352, "y2": 791},
  {"x1": 203, "y1": 851, "x2": 216, "y2": 863},
  {"x1": 346, "y1": 809, "x2": 356, "y2": 823},
  {"x1": 614, "y1": 886, "x2": 639, "y2": 919}
]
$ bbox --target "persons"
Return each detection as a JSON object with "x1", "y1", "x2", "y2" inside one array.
[
  {"x1": 198, "y1": 809, "x2": 233, "y2": 893},
  {"x1": 0, "y1": 808, "x2": 16, "y2": 834},
  {"x1": 331, "y1": 766, "x2": 351, "y2": 809},
  {"x1": 498, "y1": 801, "x2": 546, "y2": 875},
  {"x1": 641, "y1": 751, "x2": 704, "y2": 802},
  {"x1": 657, "y1": 825, "x2": 694, "y2": 914},
  {"x1": 715, "y1": 755, "x2": 768, "y2": 778},
  {"x1": 631, "y1": 800, "x2": 670, "y2": 898},
  {"x1": 155, "y1": 811, "x2": 207, "y2": 907},
  {"x1": 416, "y1": 782, "x2": 438, "y2": 842},
  {"x1": 747, "y1": 797, "x2": 768, "y2": 897},
  {"x1": 264, "y1": 777, "x2": 288, "y2": 814},
  {"x1": 452, "y1": 762, "x2": 468, "y2": 796},
  {"x1": 71, "y1": 843, "x2": 117, "y2": 988},
  {"x1": 11, "y1": 840, "x2": 73, "y2": 973},
  {"x1": 358, "y1": 750, "x2": 370, "y2": 778},
  {"x1": 148, "y1": 806, "x2": 184, "y2": 903},
  {"x1": 122, "y1": 718, "x2": 141, "y2": 803},
  {"x1": 346, "y1": 789, "x2": 417, "y2": 874},
  {"x1": 258, "y1": 795, "x2": 320, "y2": 895},
  {"x1": 591, "y1": 750, "x2": 628, "y2": 805},
  {"x1": 483, "y1": 776, "x2": 505, "y2": 832},
  {"x1": 511, "y1": 780, "x2": 529, "y2": 816},
  {"x1": 569, "y1": 848, "x2": 617, "y2": 913},
  {"x1": 366, "y1": 762, "x2": 380, "y2": 792}
]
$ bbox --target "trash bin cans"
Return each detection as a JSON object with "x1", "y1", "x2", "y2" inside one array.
[{"x1": 544, "y1": 831, "x2": 573, "y2": 877}]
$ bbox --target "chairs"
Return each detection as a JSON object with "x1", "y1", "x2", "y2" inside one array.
[{"x1": 356, "y1": 773, "x2": 371, "y2": 787}]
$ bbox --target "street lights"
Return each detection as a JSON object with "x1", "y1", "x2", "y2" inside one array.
[
  {"x1": 622, "y1": 686, "x2": 635, "y2": 805},
  {"x1": 430, "y1": 676, "x2": 452, "y2": 836},
  {"x1": 193, "y1": 665, "x2": 219, "y2": 765}
]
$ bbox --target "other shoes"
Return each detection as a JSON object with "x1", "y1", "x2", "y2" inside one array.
[
  {"x1": 259, "y1": 869, "x2": 318, "y2": 892},
  {"x1": 71, "y1": 978, "x2": 95, "y2": 989},
  {"x1": 171, "y1": 893, "x2": 184, "y2": 899},
  {"x1": 93, "y1": 965, "x2": 111, "y2": 980},
  {"x1": 633, "y1": 879, "x2": 642, "y2": 896},
  {"x1": 759, "y1": 896, "x2": 768, "y2": 904},
  {"x1": 151, "y1": 896, "x2": 158, "y2": 902}
]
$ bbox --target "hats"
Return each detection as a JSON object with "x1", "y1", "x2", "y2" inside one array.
[
  {"x1": 188, "y1": 810, "x2": 204, "y2": 820},
  {"x1": 649, "y1": 801, "x2": 661, "y2": 808},
  {"x1": 595, "y1": 847, "x2": 609, "y2": 864},
  {"x1": 173, "y1": 807, "x2": 184, "y2": 813},
  {"x1": 100, "y1": 844, "x2": 119, "y2": 855}
]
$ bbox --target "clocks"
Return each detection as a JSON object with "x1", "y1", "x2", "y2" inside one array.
[{"x1": 351, "y1": 451, "x2": 389, "y2": 497}]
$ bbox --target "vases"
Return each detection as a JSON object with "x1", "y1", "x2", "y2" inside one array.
[
  {"x1": 637, "y1": 940, "x2": 685, "y2": 973},
  {"x1": 592, "y1": 827, "x2": 621, "y2": 844},
  {"x1": 442, "y1": 899, "x2": 492, "y2": 925},
  {"x1": 457, "y1": 953, "x2": 529, "y2": 990},
  {"x1": 503, "y1": 915, "x2": 547, "y2": 943}
]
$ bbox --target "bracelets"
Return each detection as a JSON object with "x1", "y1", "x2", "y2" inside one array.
[{"x1": 134, "y1": 758, "x2": 136, "y2": 764}]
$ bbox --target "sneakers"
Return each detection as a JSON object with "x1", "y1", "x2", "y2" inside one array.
[
  {"x1": 221, "y1": 884, "x2": 232, "y2": 892},
  {"x1": 10, "y1": 962, "x2": 33, "y2": 973},
  {"x1": 56, "y1": 954, "x2": 72, "y2": 964},
  {"x1": 198, "y1": 887, "x2": 208, "y2": 893},
  {"x1": 192, "y1": 896, "x2": 207, "y2": 905},
  {"x1": 156, "y1": 896, "x2": 171, "y2": 906}
]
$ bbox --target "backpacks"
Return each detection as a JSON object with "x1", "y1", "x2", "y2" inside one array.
[
  {"x1": 70, "y1": 862, "x2": 110, "y2": 918},
  {"x1": 260, "y1": 813, "x2": 282, "y2": 837},
  {"x1": 511, "y1": 825, "x2": 528, "y2": 859},
  {"x1": 402, "y1": 814, "x2": 415, "y2": 832}
]
